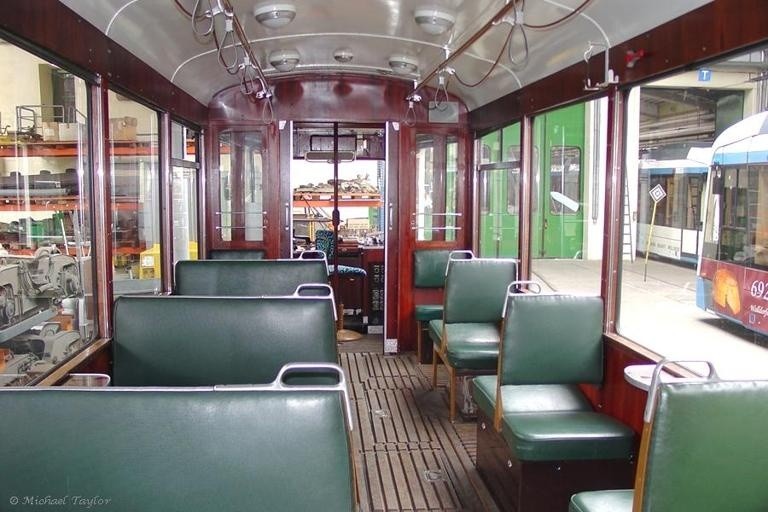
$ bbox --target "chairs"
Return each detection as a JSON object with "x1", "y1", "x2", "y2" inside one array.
[{"x1": 412, "y1": 246, "x2": 767, "y2": 510}]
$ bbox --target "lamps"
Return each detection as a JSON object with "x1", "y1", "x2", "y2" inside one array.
[
  {"x1": 413, "y1": 9, "x2": 456, "y2": 35},
  {"x1": 332, "y1": 49, "x2": 353, "y2": 64},
  {"x1": 388, "y1": 56, "x2": 418, "y2": 75},
  {"x1": 253, "y1": 3, "x2": 298, "y2": 29},
  {"x1": 267, "y1": 48, "x2": 299, "y2": 73}
]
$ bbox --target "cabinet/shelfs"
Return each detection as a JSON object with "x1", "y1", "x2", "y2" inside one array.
[{"x1": 0, "y1": 144, "x2": 230, "y2": 259}]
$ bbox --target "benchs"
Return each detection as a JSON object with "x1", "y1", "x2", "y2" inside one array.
[{"x1": 0, "y1": 247, "x2": 361, "y2": 512}]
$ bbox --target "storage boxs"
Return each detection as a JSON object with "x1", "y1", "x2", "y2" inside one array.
[
  {"x1": 59, "y1": 123, "x2": 81, "y2": 141},
  {"x1": 41, "y1": 120, "x2": 58, "y2": 141},
  {"x1": 106, "y1": 117, "x2": 139, "y2": 143}
]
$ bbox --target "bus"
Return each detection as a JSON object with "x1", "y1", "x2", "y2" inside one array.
[
  {"x1": 691, "y1": 110, "x2": 768, "y2": 340},
  {"x1": 508, "y1": 139, "x2": 718, "y2": 273}
]
